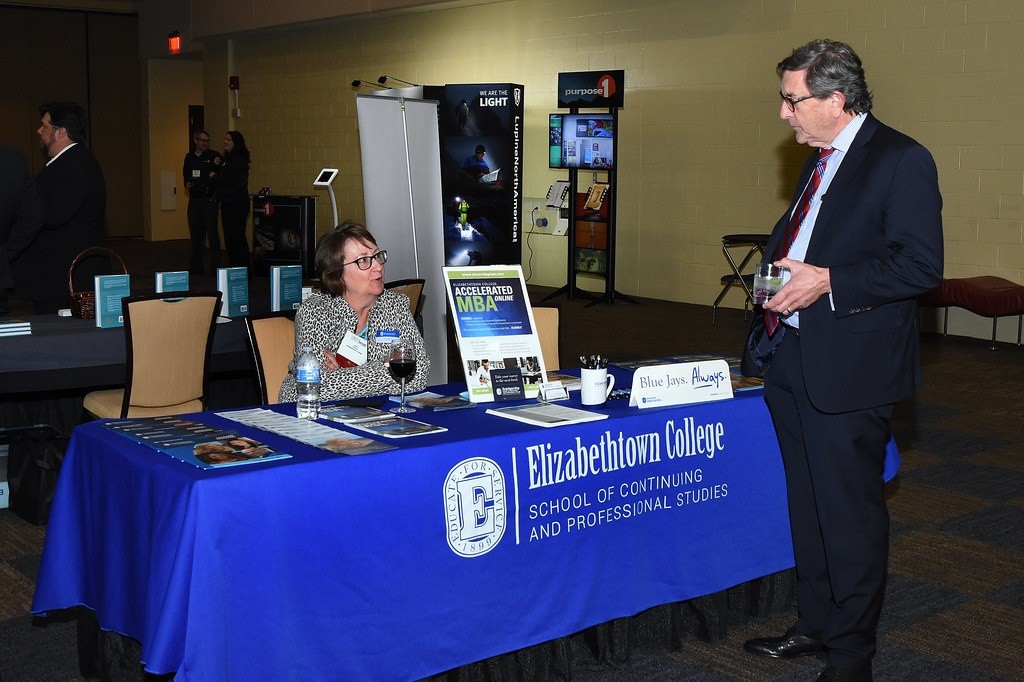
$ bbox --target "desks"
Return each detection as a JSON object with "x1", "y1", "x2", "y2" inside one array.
[
  {"x1": 31, "y1": 353, "x2": 901, "y2": 682},
  {"x1": 0, "y1": 301, "x2": 303, "y2": 481}
]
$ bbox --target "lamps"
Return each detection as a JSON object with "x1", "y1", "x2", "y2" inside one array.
[
  {"x1": 378, "y1": 76, "x2": 420, "y2": 86},
  {"x1": 351, "y1": 79, "x2": 393, "y2": 89}
]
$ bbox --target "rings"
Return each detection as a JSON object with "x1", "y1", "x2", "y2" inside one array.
[{"x1": 787, "y1": 308, "x2": 792, "y2": 314}]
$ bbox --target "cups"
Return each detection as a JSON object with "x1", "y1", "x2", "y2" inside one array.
[
  {"x1": 581, "y1": 368, "x2": 615, "y2": 405},
  {"x1": 753, "y1": 264, "x2": 784, "y2": 304}
]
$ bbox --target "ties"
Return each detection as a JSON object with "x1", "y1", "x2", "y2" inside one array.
[{"x1": 763, "y1": 146, "x2": 836, "y2": 337}]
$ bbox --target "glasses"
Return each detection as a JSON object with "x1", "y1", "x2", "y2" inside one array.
[
  {"x1": 780, "y1": 90, "x2": 825, "y2": 112},
  {"x1": 196, "y1": 139, "x2": 210, "y2": 143},
  {"x1": 342, "y1": 249, "x2": 388, "y2": 270}
]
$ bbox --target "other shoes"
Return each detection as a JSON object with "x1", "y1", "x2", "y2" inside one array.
[{"x1": 188, "y1": 268, "x2": 204, "y2": 276}]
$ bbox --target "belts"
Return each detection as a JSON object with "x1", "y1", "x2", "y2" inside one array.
[{"x1": 779, "y1": 317, "x2": 800, "y2": 336}]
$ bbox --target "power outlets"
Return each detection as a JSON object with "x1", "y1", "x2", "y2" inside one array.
[{"x1": 533, "y1": 201, "x2": 540, "y2": 211}]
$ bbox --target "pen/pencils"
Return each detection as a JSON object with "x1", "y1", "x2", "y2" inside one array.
[
  {"x1": 608, "y1": 388, "x2": 631, "y2": 400},
  {"x1": 579, "y1": 355, "x2": 608, "y2": 370}
]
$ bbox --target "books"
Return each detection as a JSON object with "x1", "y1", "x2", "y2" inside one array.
[
  {"x1": 390, "y1": 390, "x2": 477, "y2": 412},
  {"x1": 271, "y1": 266, "x2": 302, "y2": 312},
  {"x1": 102, "y1": 416, "x2": 224, "y2": 467},
  {"x1": 0, "y1": 317, "x2": 33, "y2": 337},
  {"x1": 217, "y1": 267, "x2": 249, "y2": 318},
  {"x1": 95, "y1": 274, "x2": 130, "y2": 328},
  {"x1": 485, "y1": 401, "x2": 609, "y2": 427},
  {"x1": 155, "y1": 271, "x2": 189, "y2": 293},
  {"x1": 608, "y1": 354, "x2": 764, "y2": 393},
  {"x1": 318, "y1": 404, "x2": 448, "y2": 438},
  {"x1": 547, "y1": 371, "x2": 581, "y2": 391},
  {"x1": 162, "y1": 428, "x2": 293, "y2": 470},
  {"x1": 215, "y1": 408, "x2": 399, "y2": 455}
]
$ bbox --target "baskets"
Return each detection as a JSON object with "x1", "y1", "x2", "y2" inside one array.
[{"x1": 68, "y1": 246, "x2": 134, "y2": 320}]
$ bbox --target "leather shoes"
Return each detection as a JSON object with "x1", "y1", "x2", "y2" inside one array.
[
  {"x1": 743, "y1": 623, "x2": 830, "y2": 663},
  {"x1": 815, "y1": 665, "x2": 873, "y2": 682}
]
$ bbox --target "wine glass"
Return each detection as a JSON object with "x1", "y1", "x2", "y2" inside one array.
[{"x1": 389, "y1": 341, "x2": 417, "y2": 413}]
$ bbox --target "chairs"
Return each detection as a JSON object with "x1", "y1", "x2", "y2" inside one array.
[
  {"x1": 713, "y1": 232, "x2": 772, "y2": 324},
  {"x1": 244, "y1": 308, "x2": 297, "y2": 406},
  {"x1": 384, "y1": 279, "x2": 426, "y2": 317},
  {"x1": 81, "y1": 290, "x2": 223, "y2": 422},
  {"x1": 920, "y1": 276, "x2": 1024, "y2": 350},
  {"x1": 531, "y1": 304, "x2": 560, "y2": 372}
]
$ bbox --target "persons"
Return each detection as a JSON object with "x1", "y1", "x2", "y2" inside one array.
[
  {"x1": 449, "y1": 100, "x2": 489, "y2": 231},
  {"x1": 524, "y1": 357, "x2": 542, "y2": 384},
  {"x1": 33, "y1": 99, "x2": 106, "y2": 315},
  {"x1": 278, "y1": 219, "x2": 430, "y2": 404},
  {"x1": 476, "y1": 359, "x2": 495, "y2": 388},
  {"x1": 195, "y1": 438, "x2": 276, "y2": 464},
  {"x1": 183, "y1": 130, "x2": 251, "y2": 275},
  {"x1": 0, "y1": 144, "x2": 48, "y2": 316},
  {"x1": 741, "y1": 39, "x2": 945, "y2": 682}
]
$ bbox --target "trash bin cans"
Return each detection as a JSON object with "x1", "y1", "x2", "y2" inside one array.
[{"x1": 7, "y1": 430, "x2": 72, "y2": 527}]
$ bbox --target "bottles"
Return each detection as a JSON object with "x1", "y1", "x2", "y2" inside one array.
[{"x1": 296, "y1": 347, "x2": 321, "y2": 420}]
